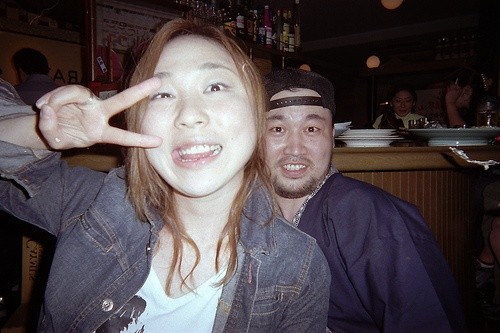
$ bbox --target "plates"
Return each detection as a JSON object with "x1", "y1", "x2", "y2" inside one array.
[
  {"x1": 407, "y1": 128, "x2": 500, "y2": 146},
  {"x1": 333, "y1": 121, "x2": 404, "y2": 148}
]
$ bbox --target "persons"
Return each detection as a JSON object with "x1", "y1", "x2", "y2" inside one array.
[
  {"x1": 444, "y1": 66, "x2": 481, "y2": 128},
  {"x1": 373, "y1": 85, "x2": 431, "y2": 129},
  {"x1": 12, "y1": 48, "x2": 65, "y2": 107},
  {"x1": 0, "y1": 18, "x2": 332, "y2": 333},
  {"x1": 257, "y1": 70, "x2": 474, "y2": 333}
]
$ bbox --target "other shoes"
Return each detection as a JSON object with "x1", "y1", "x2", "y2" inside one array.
[{"x1": 474, "y1": 262, "x2": 496, "y2": 299}]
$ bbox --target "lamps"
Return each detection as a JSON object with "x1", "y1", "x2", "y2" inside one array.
[
  {"x1": 366, "y1": 56, "x2": 380, "y2": 123},
  {"x1": 301, "y1": 65, "x2": 311, "y2": 71},
  {"x1": 382, "y1": 0, "x2": 404, "y2": 10}
]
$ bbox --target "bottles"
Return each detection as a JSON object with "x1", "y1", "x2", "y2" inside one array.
[
  {"x1": 476, "y1": 100, "x2": 497, "y2": 144},
  {"x1": 160, "y1": 0, "x2": 303, "y2": 55}
]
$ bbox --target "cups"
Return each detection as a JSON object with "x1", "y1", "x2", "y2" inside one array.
[{"x1": 408, "y1": 118, "x2": 426, "y2": 142}]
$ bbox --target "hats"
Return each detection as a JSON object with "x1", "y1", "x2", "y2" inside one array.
[{"x1": 259, "y1": 67, "x2": 337, "y2": 114}]
